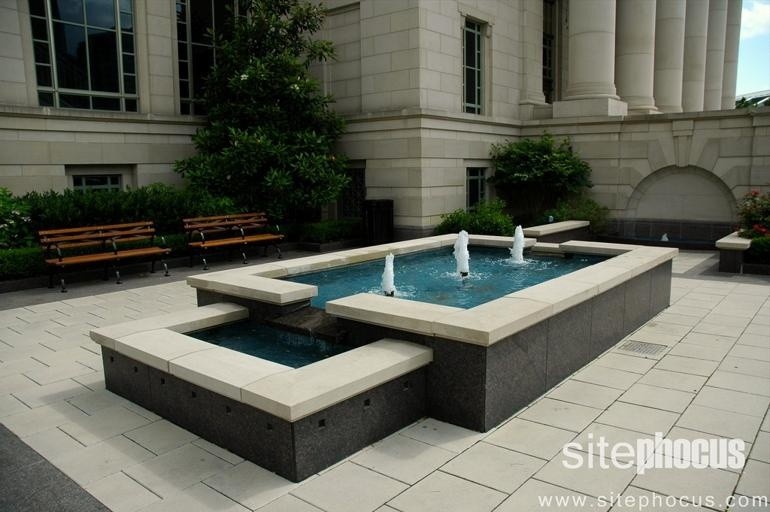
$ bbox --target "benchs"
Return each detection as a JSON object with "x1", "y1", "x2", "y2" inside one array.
[
  {"x1": 183, "y1": 213, "x2": 285, "y2": 270},
  {"x1": 38, "y1": 222, "x2": 172, "y2": 293}
]
